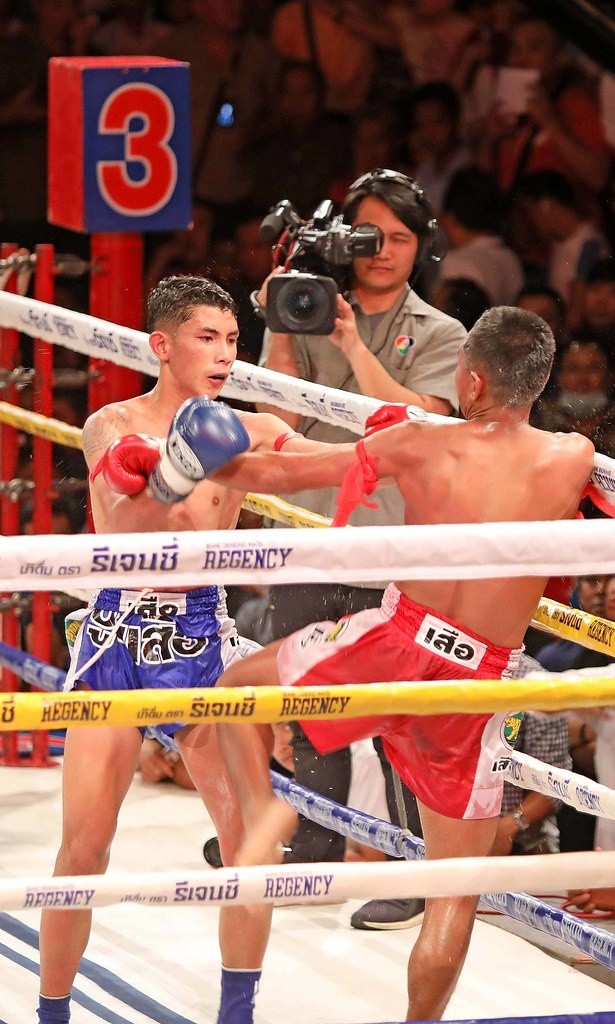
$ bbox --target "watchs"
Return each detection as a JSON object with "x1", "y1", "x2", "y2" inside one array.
[{"x1": 508, "y1": 810, "x2": 529, "y2": 832}]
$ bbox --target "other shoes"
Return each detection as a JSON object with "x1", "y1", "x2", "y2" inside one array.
[
  {"x1": 351, "y1": 898, "x2": 425, "y2": 930},
  {"x1": 271, "y1": 852, "x2": 349, "y2": 907}
]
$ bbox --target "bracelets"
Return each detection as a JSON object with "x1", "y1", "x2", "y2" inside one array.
[{"x1": 520, "y1": 802, "x2": 532, "y2": 824}]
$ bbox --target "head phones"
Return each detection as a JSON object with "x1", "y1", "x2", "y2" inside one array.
[{"x1": 345, "y1": 168, "x2": 447, "y2": 277}]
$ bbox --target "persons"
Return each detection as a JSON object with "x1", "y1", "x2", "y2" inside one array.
[
  {"x1": 204, "y1": 173, "x2": 470, "y2": 930},
  {"x1": 36, "y1": 276, "x2": 357, "y2": 1024},
  {"x1": 104, "y1": 308, "x2": 594, "y2": 1024},
  {"x1": 0, "y1": 0, "x2": 615, "y2": 913}
]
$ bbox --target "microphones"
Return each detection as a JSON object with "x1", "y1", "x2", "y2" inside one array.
[{"x1": 259, "y1": 200, "x2": 292, "y2": 240}]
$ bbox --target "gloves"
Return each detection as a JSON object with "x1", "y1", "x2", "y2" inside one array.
[
  {"x1": 92, "y1": 433, "x2": 161, "y2": 495},
  {"x1": 362, "y1": 401, "x2": 426, "y2": 438},
  {"x1": 148, "y1": 394, "x2": 251, "y2": 505}
]
